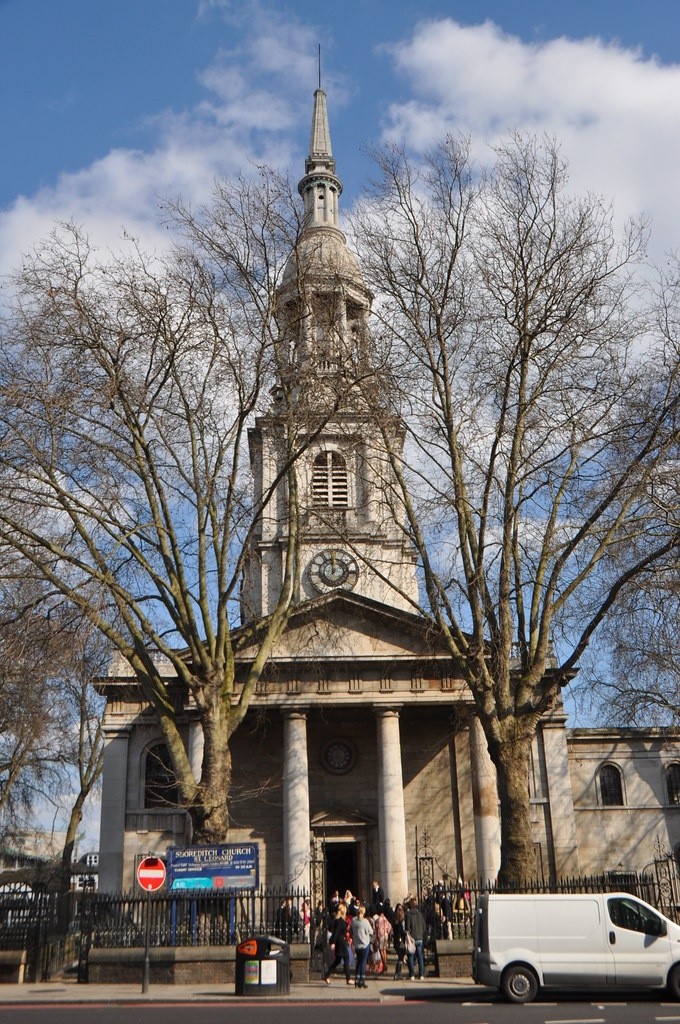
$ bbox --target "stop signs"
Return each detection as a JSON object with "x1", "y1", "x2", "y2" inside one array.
[{"x1": 137, "y1": 856, "x2": 168, "y2": 892}]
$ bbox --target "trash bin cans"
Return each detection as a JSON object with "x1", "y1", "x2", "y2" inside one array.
[{"x1": 234, "y1": 935, "x2": 291, "y2": 996}]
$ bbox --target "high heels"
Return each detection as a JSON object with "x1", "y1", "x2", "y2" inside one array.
[
  {"x1": 378, "y1": 966, "x2": 387, "y2": 976},
  {"x1": 359, "y1": 978, "x2": 368, "y2": 988},
  {"x1": 355, "y1": 977, "x2": 359, "y2": 988}
]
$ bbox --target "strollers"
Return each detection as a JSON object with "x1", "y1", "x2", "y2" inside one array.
[{"x1": 367, "y1": 942, "x2": 388, "y2": 975}]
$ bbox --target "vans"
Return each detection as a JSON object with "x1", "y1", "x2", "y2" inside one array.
[{"x1": 471, "y1": 892, "x2": 680, "y2": 1005}]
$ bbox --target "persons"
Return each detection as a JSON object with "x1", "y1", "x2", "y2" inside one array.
[{"x1": 277, "y1": 880, "x2": 470, "y2": 989}]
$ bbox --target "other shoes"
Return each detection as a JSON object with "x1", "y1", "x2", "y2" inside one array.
[
  {"x1": 406, "y1": 976, "x2": 414, "y2": 981},
  {"x1": 323, "y1": 976, "x2": 331, "y2": 984},
  {"x1": 394, "y1": 972, "x2": 404, "y2": 980},
  {"x1": 347, "y1": 980, "x2": 355, "y2": 984},
  {"x1": 418, "y1": 975, "x2": 425, "y2": 980}
]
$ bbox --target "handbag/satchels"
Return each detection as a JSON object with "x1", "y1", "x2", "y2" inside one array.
[
  {"x1": 372, "y1": 949, "x2": 381, "y2": 962},
  {"x1": 405, "y1": 931, "x2": 416, "y2": 953}
]
